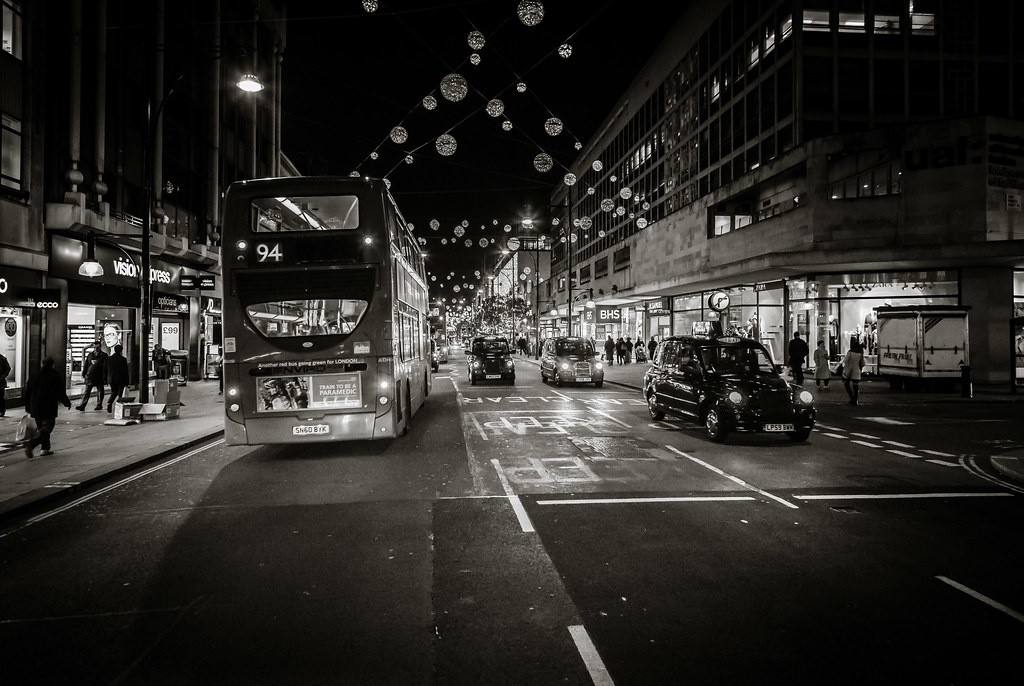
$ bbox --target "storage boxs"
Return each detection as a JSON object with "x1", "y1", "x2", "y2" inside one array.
[{"x1": 114, "y1": 378, "x2": 185, "y2": 421}]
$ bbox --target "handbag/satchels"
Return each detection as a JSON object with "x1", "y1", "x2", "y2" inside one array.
[
  {"x1": 842, "y1": 363, "x2": 853, "y2": 379},
  {"x1": 15, "y1": 414, "x2": 39, "y2": 444}
]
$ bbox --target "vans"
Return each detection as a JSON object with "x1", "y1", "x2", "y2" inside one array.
[{"x1": 540, "y1": 336, "x2": 604, "y2": 387}]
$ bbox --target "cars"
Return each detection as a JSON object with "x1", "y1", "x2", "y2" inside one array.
[{"x1": 429, "y1": 302, "x2": 448, "y2": 372}]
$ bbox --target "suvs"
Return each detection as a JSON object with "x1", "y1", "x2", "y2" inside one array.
[
  {"x1": 643, "y1": 337, "x2": 816, "y2": 443},
  {"x1": 465, "y1": 336, "x2": 516, "y2": 386}
]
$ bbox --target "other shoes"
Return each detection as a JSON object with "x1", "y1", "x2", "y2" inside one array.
[
  {"x1": 24, "y1": 446, "x2": 34, "y2": 458},
  {"x1": 816, "y1": 387, "x2": 831, "y2": 392},
  {"x1": 76, "y1": 406, "x2": 85, "y2": 411},
  {"x1": 0, "y1": 410, "x2": 6, "y2": 417},
  {"x1": 40, "y1": 450, "x2": 54, "y2": 456},
  {"x1": 107, "y1": 404, "x2": 112, "y2": 413},
  {"x1": 848, "y1": 400, "x2": 858, "y2": 406},
  {"x1": 95, "y1": 405, "x2": 102, "y2": 410}
]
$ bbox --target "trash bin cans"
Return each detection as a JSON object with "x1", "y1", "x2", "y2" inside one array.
[{"x1": 153, "y1": 378, "x2": 180, "y2": 418}]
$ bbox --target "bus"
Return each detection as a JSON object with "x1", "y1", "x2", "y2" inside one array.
[{"x1": 220, "y1": 177, "x2": 432, "y2": 445}]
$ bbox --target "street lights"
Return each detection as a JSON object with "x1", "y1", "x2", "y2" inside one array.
[
  {"x1": 509, "y1": 234, "x2": 539, "y2": 360},
  {"x1": 541, "y1": 186, "x2": 571, "y2": 338},
  {"x1": 77, "y1": 230, "x2": 153, "y2": 404},
  {"x1": 237, "y1": 44, "x2": 283, "y2": 174}
]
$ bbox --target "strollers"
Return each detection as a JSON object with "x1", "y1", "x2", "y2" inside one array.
[{"x1": 635, "y1": 346, "x2": 648, "y2": 363}]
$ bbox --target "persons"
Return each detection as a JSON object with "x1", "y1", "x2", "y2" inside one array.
[
  {"x1": 829, "y1": 307, "x2": 879, "y2": 362},
  {"x1": 788, "y1": 332, "x2": 809, "y2": 385},
  {"x1": 0, "y1": 342, "x2": 223, "y2": 459},
  {"x1": 518, "y1": 337, "x2": 552, "y2": 358},
  {"x1": 843, "y1": 338, "x2": 865, "y2": 406},
  {"x1": 103, "y1": 323, "x2": 122, "y2": 356},
  {"x1": 592, "y1": 335, "x2": 657, "y2": 366},
  {"x1": 814, "y1": 341, "x2": 831, "y2": 392},
  {"x1": 730, "y1": 314, "x2": 758, "y2": 339}
]
